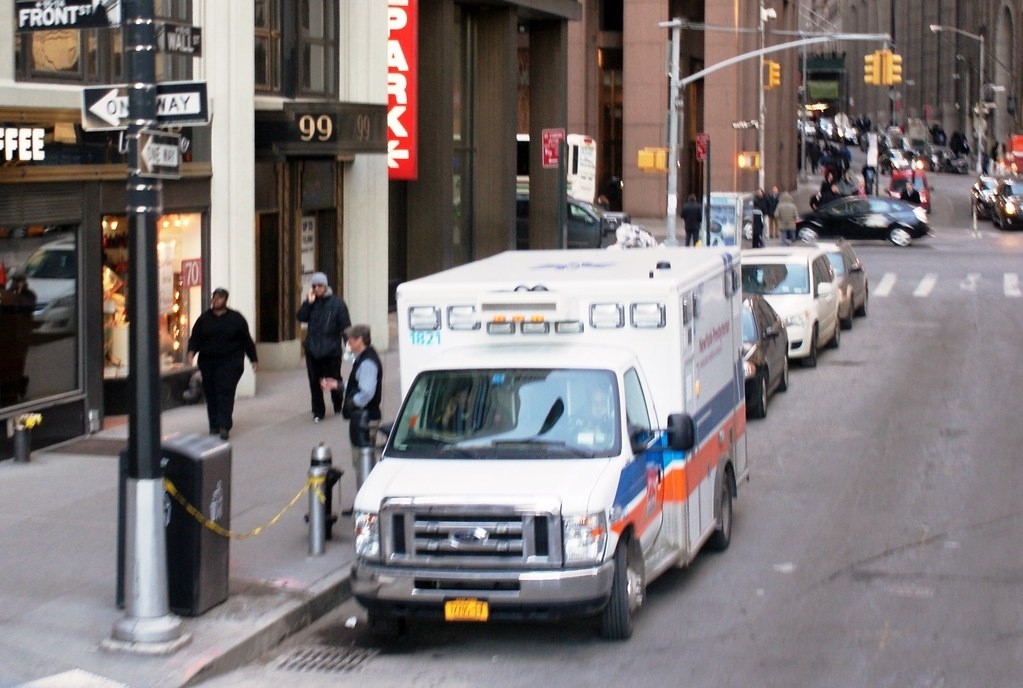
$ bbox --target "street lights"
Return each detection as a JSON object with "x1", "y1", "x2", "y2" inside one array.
[{"x1": 931, "y1": 26, "x2": 984, "y2": 179}]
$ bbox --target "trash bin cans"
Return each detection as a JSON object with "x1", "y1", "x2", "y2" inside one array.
[
  {"x1": 117, "y1": 439, "x2": 232, "y2": 615},
  {"x1": 599, "y1": 211, "x2": 631, "y2": 250}
]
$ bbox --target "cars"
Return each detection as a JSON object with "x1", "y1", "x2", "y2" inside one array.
[
  {"x1": 742, "y1": 291, "x2": 788, "y2": 419},
  {"x1": 8, "y1": 238, "x2": 77, "y2": 336},
  {"x1": 799, "y1": 117, "x2": 860, "y2": 144},
  {"x1": 517, "y1": 192, "x2": 631, "y2": 247},
  {"x1": 791, "y1": 238, "x2": 869, "y2": 330},
  {"x1": 797, "y1": 193, "x2": 930, "y2": 248},
  {"x1": 860, "y1": 131, "x2": 968, "y2": 175},
  {"x1": 885, "y1": 169, "x2": 934, "y2": 211}
]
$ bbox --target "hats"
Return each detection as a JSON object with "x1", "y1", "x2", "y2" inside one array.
[
  {"x1": 11, "y1": 272, "x2": 27, "y2": 280},
  {"x1": 311, "y1": 272, "x2": 328, "y2": 286},
  {"x1": 344, "y1": 323, "x2": 371, "y2": 336}
]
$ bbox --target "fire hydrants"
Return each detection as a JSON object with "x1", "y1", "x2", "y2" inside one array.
[{"x1": 303, "y1": 443, "x2": 342, "y2": 541}]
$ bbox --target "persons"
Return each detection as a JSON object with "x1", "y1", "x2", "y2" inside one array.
[
  {"x1": 103, "y1": 252, "x2": 125, "y2": 365},
  {"x1": 319, "y1": 324, "x2": 383, "y2": 515},
  {"x1": 186, "y1": 288, "x2": 259, "y2": 438},
  {"x1": 599, "y1": 111, "x2": 1005, "y2": 248},
  {"x1": 1, "y1": 271, "x2": 36, "y2": 404},
  {"x1": 296, "y1": 273, "x2": 352, "y2": 421}
]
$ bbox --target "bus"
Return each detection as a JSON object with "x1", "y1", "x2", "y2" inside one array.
[{"x1": 514, "y1": 133, "x2": 598, "y2": 204}]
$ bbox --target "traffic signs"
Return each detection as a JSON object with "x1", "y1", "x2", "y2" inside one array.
[
  {"x1": 79, "y1": 80, "x2": 210, "y2": 133},
  {"x1": 135, "y1": 131, "x2": 180, "y2": 180},
  {"x1": 162, "y1": 20, "x2": 202, "y2": 57}
]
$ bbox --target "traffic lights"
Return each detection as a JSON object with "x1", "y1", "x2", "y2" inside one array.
[
  {"x1": 864, "y1": 50, "x2": 881, "y2": 86},
  {"x1": 770, "y1": 62, "x2": 780, "y2": 88},
  {"x1": 656, "y1": 150, "x2": 669, "y2": 169},
  {"x1": 886, "y1": 51, "x2": 903, "y2": 85},
  {"x1": 739, "y1": 152, "x2": 759, "y2": 168}
]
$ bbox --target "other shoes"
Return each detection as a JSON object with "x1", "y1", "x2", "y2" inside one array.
[
  {"x1": 341, "y1": 507, "x2": 353, "y2": 516},
  {"x1": 209, "y1": 428, "x2": 219, "y2": 436},
  {"x1": 219, "y1": 427, "x2": 229, "y2": 440}
]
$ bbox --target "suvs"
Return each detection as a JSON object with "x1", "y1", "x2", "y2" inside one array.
[{"x1": 971, "y1": 173, "x2": 1023, "y2": 231}]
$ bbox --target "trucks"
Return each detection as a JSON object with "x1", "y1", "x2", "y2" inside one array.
[{"x1": 348, "y1": 248, "x2": 751, "y2": 643}]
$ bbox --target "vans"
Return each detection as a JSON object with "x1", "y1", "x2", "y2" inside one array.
[{"x1": 742, "y1": 246, "x2": 841, "y2": 365}]
